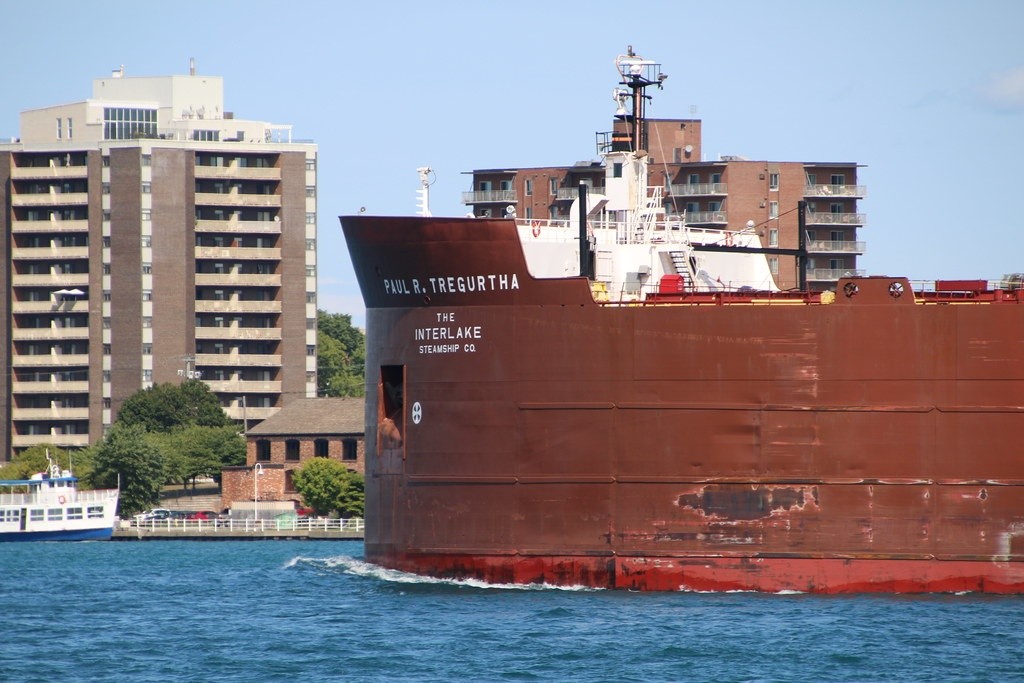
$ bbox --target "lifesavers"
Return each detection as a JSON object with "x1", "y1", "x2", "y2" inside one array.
[
  {"x1": 58, "y1": 495, "x2": 66, "y2": 504},
  {"x1": 532, "y1": 222, "x2": 541, "y2": 237},
  {"x1": 725, "y1": 232, "x2": 733, "y2": 247}
]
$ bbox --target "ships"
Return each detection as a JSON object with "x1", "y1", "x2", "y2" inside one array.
[{"x1": 335, "y1": 44, "x2": 1023, "y2": 595}]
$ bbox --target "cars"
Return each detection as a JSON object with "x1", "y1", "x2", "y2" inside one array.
[{"x1": 130, "y1": 508, "x2": 221, "y2": 527}]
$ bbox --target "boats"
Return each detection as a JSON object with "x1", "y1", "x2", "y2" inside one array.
[{"x1": 0, "y1": 447, "x2": 121, "y2": 542}]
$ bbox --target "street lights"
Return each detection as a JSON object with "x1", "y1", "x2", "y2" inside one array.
[{"x1": 254, "y1": 462, "x2": 263, "y2": 520}]
